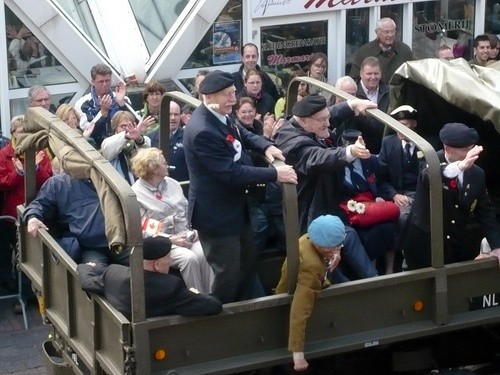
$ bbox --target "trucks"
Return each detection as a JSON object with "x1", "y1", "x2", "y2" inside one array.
[{"x1": 17, "y1": 74, "x2": 499, "y2": 375}]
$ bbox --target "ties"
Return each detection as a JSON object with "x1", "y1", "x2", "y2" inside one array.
[
  {"x1": 348, "y1": 164, "x2": 368, "y2": 193},
  {"x1": 405, "y1": 144, "x2": 412, "y2": 161}
]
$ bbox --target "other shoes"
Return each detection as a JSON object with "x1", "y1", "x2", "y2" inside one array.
[{"x1": 15, "y1": 292, "x2": 28, "y2": 313}]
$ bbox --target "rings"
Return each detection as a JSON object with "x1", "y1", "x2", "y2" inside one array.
[{"x1": 359, "y1": 153, "x2": 361, "y2": 156}]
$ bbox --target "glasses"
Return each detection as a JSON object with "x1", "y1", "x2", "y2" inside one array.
[{"x1": 309, "y1": 114, "x2": 331, "y2": 122}]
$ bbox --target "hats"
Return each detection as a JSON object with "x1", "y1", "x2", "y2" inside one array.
[
  {"x1": 440, "y1": 123, "x2": 479, "y2": 148},
  {"x1": 308, "y1": 214, "x2": 345, "y2": 248},
  {"x1": 291, "y1": 93, "x2": 326, "y2": 118},
  {"x1": 198, "y1": 69, "x2": 236, "y2": 94},
  {"x1": 342, "y1": 129, "x2": 363, "y2": 141},
  {"x1": 390, "y1": 105, "x2": 417, "y2": 121},
  {"x1": 142, "y1": 236, "x2": 171, "y2": 260}
]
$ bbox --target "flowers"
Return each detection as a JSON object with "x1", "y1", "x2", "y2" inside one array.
[
  {"x1": 226, "y1": 134, "x2": 233, "y2": 142},
  {"x1": 347, "y1": 199, "x2": 366, "y2": 213},
  {"x1": 448, "y1": 179, "x2": 457, "y2": 188}
]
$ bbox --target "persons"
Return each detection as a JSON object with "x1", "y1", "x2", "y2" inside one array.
[
  {"x1": 275, "y1": 214, "x2": 346, "y2": 370},
  {"x1": 353, "y1": 18, "x2": 416, "y2": 83},
  {"x1": 0, "y1": 34, "x2": 500, "y2": 320},
  {"x1": 6, "y1": 25, "x2": 39, "y2": 72}
]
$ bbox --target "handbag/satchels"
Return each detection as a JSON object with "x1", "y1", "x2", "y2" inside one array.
[{"x1": 342, "y1": 192, "x2": 401, "y2": 226}]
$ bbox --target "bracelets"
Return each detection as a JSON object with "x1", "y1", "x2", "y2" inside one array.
[{"x1": 135, "y1": 136, "x2": 142, "y2": 143}]
